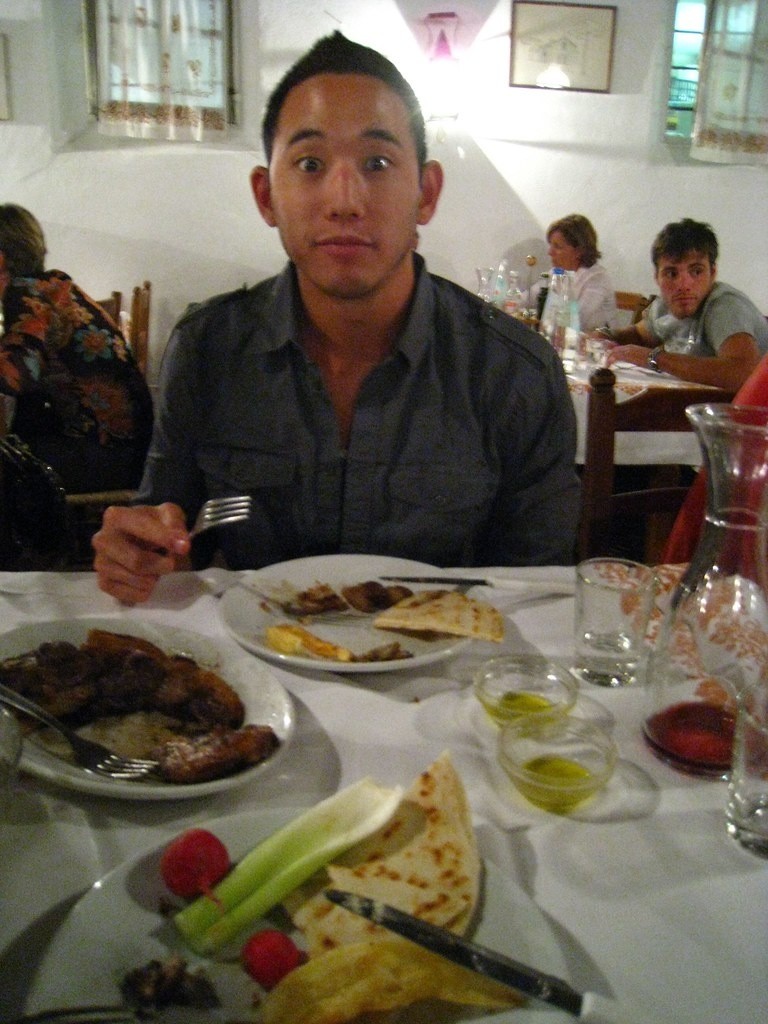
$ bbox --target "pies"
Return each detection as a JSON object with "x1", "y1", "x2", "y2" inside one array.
[
  {"x1": 281, "y1": 750, "x2": 480, "y2": 952},
  {"x1": 247, "y1": 937, "x2": 530, "y2": 1024},
  {"x1": 375, "y1": 589, "x2": 507, "y2": 641},
  {"x1": 265, "y1": 623, "x2": 354, "y2": 662}
]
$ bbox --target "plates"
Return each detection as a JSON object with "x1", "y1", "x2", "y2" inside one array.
[
  {"x1": 1, "y1": 617, "x2": 298, "y2": 798},
  {"x1": 217, "y1": 553, "x2": 468, "y2": 674},
  {"x1": 22, "y1": 809, "x2": 571, "y2": 1024}
]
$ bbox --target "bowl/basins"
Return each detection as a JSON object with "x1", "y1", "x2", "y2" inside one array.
[
  {"x1": 497, "y1": 714, "x2": 615, "y2": 812},
  {"x1": 473, "y1": 655, "x2": 580, "y2": 735}
]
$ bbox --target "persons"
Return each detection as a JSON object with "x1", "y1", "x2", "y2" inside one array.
[
  {"x1": 89, "y1": 29, "x2": 584, "y2": 616},
  {"x1": 540, "y1": 213, "x2": 619, "y2": 353},
  {"x1": 590, "y1": 218, "x2": 768, "y2": 394},
  {"x1": 0, "y1": 196, "x2": 155, "y2": 574}
]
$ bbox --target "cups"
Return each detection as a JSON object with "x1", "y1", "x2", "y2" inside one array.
[
  {"x1": 570, "y1": 557, "x2": 657, "y2": 687},
  {"x1": 724, "y1": 684, "x2": 768, "y2": 857},
  {"x1": 586, "y1": 338, "x2": 609, "y2": 372}
]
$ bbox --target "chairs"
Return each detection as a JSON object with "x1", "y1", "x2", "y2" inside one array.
[
  {"x1": 127, "y1": 281, "x2": 152, "y2": 374},
  {"x1": 578, "y1": 370, "x2": 735, "y2": 569}
]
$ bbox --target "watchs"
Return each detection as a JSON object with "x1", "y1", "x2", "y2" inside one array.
[{"x1": 644, "y1": 345, "x2": 669, "y2": 378}]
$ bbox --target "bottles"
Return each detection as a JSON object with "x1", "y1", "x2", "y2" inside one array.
[
  {"x1": 636, "y1": 402, "x2": 768, "y2": 777},
  {"x1": 475, "y1": 258, "x2": 578, "y2": 374}
]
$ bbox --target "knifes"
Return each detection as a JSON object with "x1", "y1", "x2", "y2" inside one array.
[
  {"x1": 378, "y1": 573, "x2": 574, "y2": 600},
  {"x1": 325, "y1": 888, "x2": 650, "y2": 1024}
]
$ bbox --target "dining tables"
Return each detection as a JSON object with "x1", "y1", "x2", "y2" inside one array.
[
  {"x1": 548, "y1": 333, "x2": 721, "y2": 554},
  {"x1": 0, "y1": 568, "x2": 768, "y2": 1024}
]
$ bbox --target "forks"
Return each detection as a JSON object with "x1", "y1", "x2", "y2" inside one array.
[
  {"x1": 0, "y1": 683, "x2": 158, "y2": 777},
  {"x1": 184, "y1": 495, "x2": 251, "y2": 541}
]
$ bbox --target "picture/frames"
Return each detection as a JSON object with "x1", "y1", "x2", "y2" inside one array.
[{"x1": 509, "y1": 0, "x2": 616, "y2": 94}]
